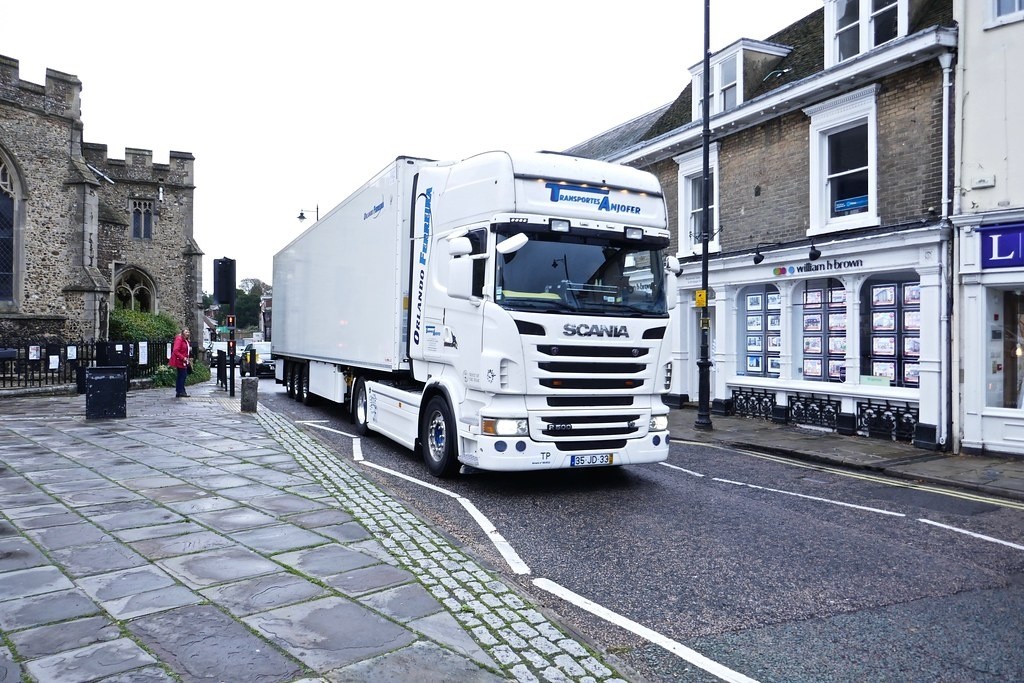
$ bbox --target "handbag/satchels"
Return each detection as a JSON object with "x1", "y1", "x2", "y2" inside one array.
[{"x1": 187, "y1": 362, "x2": 192, "y2": 374}]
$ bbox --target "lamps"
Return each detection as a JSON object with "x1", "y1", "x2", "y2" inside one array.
[
  {"x1": 809, "y1": 233, "x2": 847, "y2": 262},
  {"x1": 753, "y1": 240, "x2": 782, "y2": 266}
]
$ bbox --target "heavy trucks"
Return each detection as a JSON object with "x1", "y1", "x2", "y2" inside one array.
[{"x1": 268, "y1": 148, "x2": 688, "y2": 477}]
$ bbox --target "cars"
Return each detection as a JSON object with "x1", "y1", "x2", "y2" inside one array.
[
  {"x1": 211, "y1": 348, "x2": 240, "y2": 368},
  {"x1": 240, "y1": 341, "x2": 276, "y2": 378}
]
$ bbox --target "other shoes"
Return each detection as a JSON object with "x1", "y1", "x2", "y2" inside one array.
[{"x1": 176, "y1": 393, "x2": 190, "y2": 397}]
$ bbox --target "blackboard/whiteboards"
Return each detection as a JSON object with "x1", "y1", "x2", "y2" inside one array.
[{"x1": 216, "y1": 349, "x2": 228, "y2": 391}]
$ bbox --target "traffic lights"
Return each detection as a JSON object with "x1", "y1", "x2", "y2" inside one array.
[
  {"x1": 226, "y1": 315, "x2": 236, "y2": 329},
  {"x1": 227, "y1": 340, "x2": 236, "y2": 355}
]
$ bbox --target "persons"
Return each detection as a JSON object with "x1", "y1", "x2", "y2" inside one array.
[{"x1": 167, "y1": 327, "x2": 194, "y2": 397}]
